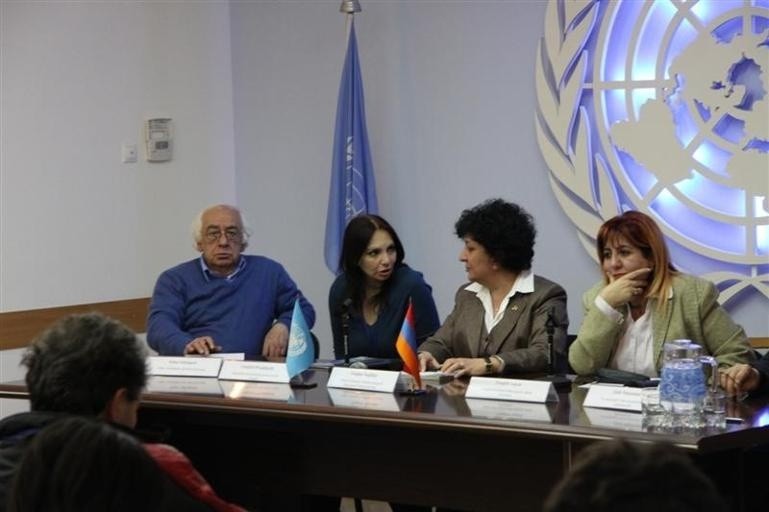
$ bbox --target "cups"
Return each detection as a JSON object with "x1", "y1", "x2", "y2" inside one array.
[{"x1": 642, "y1": 387, "x2": 726, "y2": 429}]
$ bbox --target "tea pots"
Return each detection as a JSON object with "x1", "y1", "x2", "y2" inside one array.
[{"x1": 660, "y1": 338, "x2": 717, "y2": 413}]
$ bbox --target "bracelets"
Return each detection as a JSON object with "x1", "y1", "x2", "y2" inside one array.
[{"x1": 484, "y1": 355, "x2": 492, "y2": 372}]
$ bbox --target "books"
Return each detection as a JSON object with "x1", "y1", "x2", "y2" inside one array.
[
  {"x1": 187, "y1": 353, "x2": 246, "y2": 361},
  {"x1": 418, "y1": 370, "x2": 450, "y2": 378}
]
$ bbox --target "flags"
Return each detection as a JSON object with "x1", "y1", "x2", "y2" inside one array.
[
  {"x1": 286, "y1": 303, "x2": 314, "y2": 382},
  {"x1": 394, "y1": 304, "x2": 423, "y2": 391},
  {"x1": 323, "y1": 1, "x2": 379, "y2": 275}
]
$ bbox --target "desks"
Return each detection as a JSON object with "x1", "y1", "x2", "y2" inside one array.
[{"x1": 0, "y1": 354, "x2": 769, "y2": 512}]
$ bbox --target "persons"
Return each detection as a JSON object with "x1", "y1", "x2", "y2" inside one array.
[
  {"x1": 146, "y1": 202, "x2": 317, "y2": 357},
  {"x1": 417, "y1": 198, "x2": 569, "y2": 378},
  {"x1": 719, "y1": 350, "x2": 769, "y2": 404},
  {"x1": 327, "y1": 214, "x2": 442, "y2": 371},
  {"x1": 567, "y1": 211, "x2": 756, "y2": 389},
  {"x1": 0, "y1": 311, "x2": 215, "y2": 512}
]
